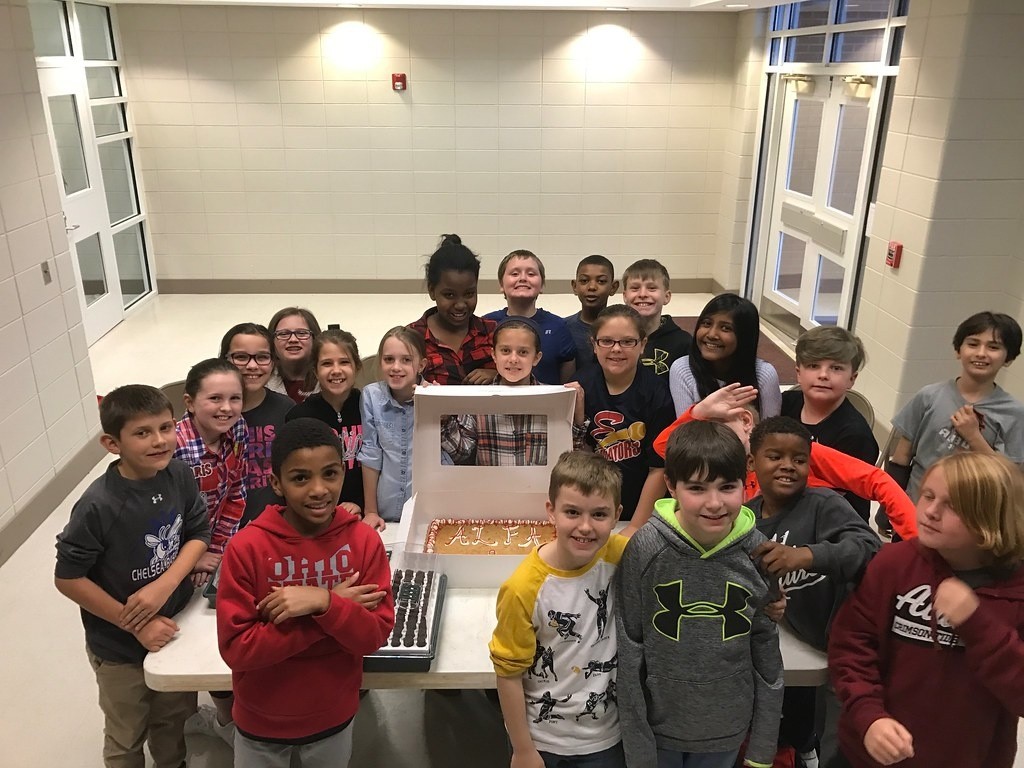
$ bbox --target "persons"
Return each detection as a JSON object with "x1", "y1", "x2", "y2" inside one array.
[
  {"x1": 488, "y1": 450, "x2": 786, "y2": 768},
  {"x1": 171, "y1": 359, "x2": 250, "y2": 751},
  {"x1": 479, "y1": 250, "x2": 576, "y2": 386},
  {"x1": 216, "y1": 418, "x2": 394, "y2": 768},
  {"x1": 652, "y1": 383, "x2": 920, "y2": 542},
  {"x1": 613, "y1": 418, "x2": 785, "y2": 768},
  {"x1": 54, "y1": 385, "x2": 211, "y2": 768},
  {"x1": 827, "y1": 453, "x2": 1024, "y2": 768},
  {"x1": 568, "y1": 255, "x2": 1024, "y2": 538},
  {"x1": 264, "y1": 307, "x2": 322, "y2": 404},
  {"x1": 284, "y1": 324, "x2": 366, "y2": 520},
  {"x1": 356, "y1": 325, "x2": 455, "y2": 531},
  {"x1": 218, "y1": 322, "x2": 297, "y2": 532},
  {"x1": 411, "y1": 316, "x2": 590, "y2": 467},
  {"x1": 403, "y1": 233, "x2": 499, "y2": 385},
  {"x1": 742, "y1": 415, "x2": 884, "y2": 768}
]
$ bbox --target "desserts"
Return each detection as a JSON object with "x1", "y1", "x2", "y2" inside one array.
[{"x1": 372, "y1": 569, "x2": 441, "y2": 653}]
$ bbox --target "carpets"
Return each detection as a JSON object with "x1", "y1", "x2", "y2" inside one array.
[{"x1": 670, "y1": 317, "x2": 798, "y2": 385}]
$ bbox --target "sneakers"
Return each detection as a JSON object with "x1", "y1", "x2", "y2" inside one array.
[{"x1": 213, "y1": 714, "x2": 236, "y2": 749}]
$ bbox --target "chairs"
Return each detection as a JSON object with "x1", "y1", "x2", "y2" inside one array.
[
  {"x1": 789, "y1": 383, "x2": 874, "y2": 430},
  {"x1": 159, "y1": 379, "x2": 187, "y2": 421},
  {"x1": 875, "y1": 426, "x2": 902, "y2": 471},
  {"x1": 353, "y1": 355, "x2": 386, "y2": 393}
]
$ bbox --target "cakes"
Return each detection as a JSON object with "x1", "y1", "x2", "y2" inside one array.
[{"x1": 424, "y1": 518, "x2": 559, "y2": 555}]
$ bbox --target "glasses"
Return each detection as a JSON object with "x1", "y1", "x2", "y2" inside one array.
[
  {"x1": 225, "y1": 351, "x2": 273, "y2": 365},
  {"x1": 273, "y1": 329, "x2": 315, "y2": 340},
  {"x1": 594, "y1": 338, "x2": 643, "y2": 348}
]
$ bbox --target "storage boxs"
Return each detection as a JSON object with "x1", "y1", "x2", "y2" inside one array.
[{"x1": 405, "y1": 385, "x2": 577, "y2": 589}]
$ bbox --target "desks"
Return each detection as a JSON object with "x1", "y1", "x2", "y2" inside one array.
[{"x1": 144, "y1": 523, "x2": 830, "y2": 768}]
